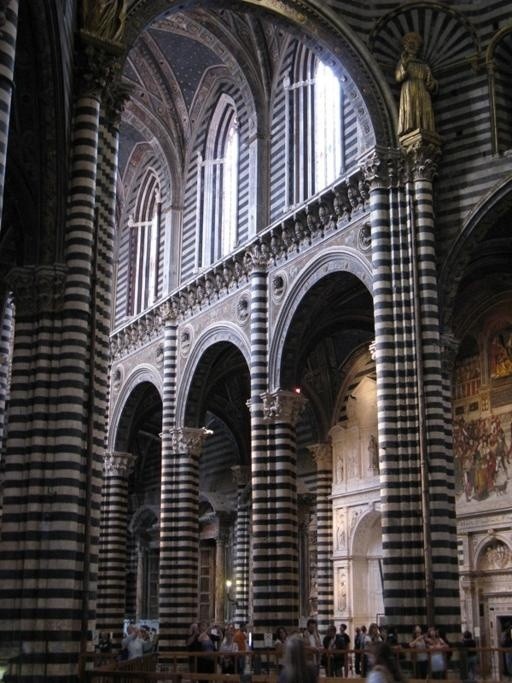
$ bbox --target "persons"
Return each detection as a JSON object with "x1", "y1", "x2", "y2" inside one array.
[
  {"x1": 273, "y1": 619, "x2": 481, "y2": 683},
  {"x1": 501, "y1": 623, "x2": 512, "y2": 680},
  {"x1": 121, "y1": 624, "x2": 159, "y2": 660},
  {"x1": 190, "y1": 617, "x2": 251, "y2": 675}
]
